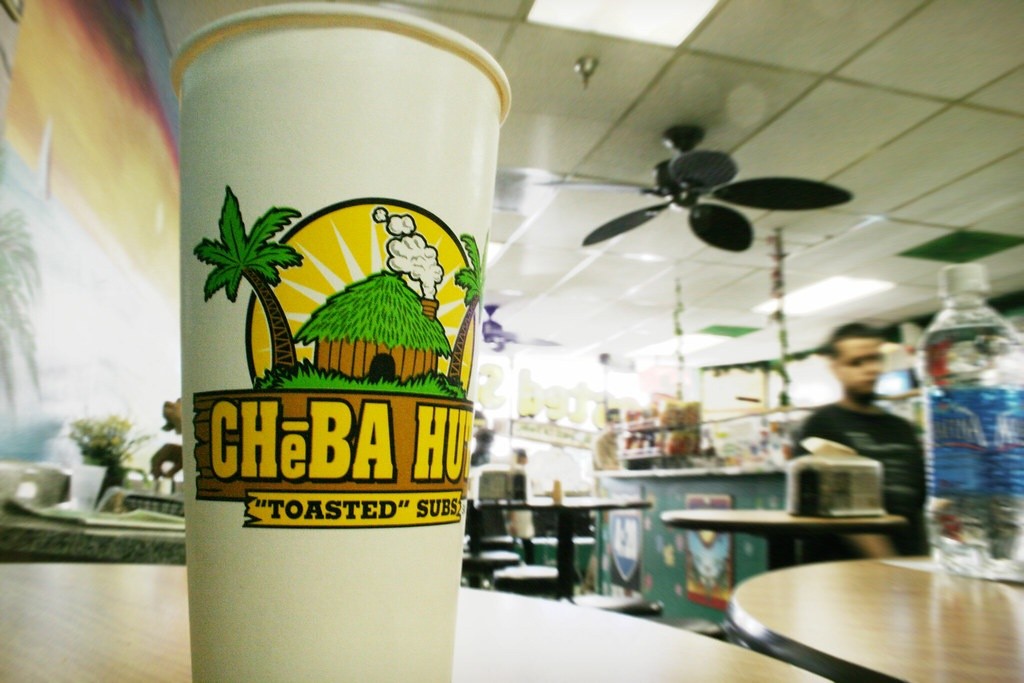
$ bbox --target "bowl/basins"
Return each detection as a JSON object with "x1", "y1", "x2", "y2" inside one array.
[{"x1": 1, "y1": 461, "x2": 25, "y2": 510}]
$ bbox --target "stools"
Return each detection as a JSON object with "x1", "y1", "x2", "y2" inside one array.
[{"x1": 454, "y1": 501, "x2": 729, "y2": 638}]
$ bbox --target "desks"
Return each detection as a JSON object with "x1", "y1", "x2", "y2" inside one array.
[
  {"x1": 487, "y1": 497, "x2": 1023, "y2": 683},
  {"x1": 0, "y1": 543, "x2": 834, "y2": 682}
]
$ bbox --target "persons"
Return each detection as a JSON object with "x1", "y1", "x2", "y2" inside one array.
[
  {"x1": 791, "y1": 322, "x2": 931, "y2": 564},
  {"x1": 591, "y1": 409, "x2": 621, "y2": 470},
  {"x1": 471, "y1": 429, "x2": 493, "y2": 467},
  {"x1": 513, "y1": 449, "x2": 527, "y2": 470}
]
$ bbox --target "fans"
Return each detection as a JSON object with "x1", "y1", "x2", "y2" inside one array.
[{"x1": 534, "y1": 124, "x2": 853, "y2": 254}]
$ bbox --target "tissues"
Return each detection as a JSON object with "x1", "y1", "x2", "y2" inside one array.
[{"x1": 785, "y1": 437, "x2": 889, "y2": 518}]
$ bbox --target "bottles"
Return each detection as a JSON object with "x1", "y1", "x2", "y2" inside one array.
[{"x1": 917, "y1": 261, "x2": 1024, "y2": 583}]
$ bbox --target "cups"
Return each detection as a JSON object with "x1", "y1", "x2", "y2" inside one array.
[
  {"x1": 166, "y1": 0, "x2": 513, "y2": 683},
  {"x1": 69, "y1": 464, "x2": 108, "y2": 514}
]
